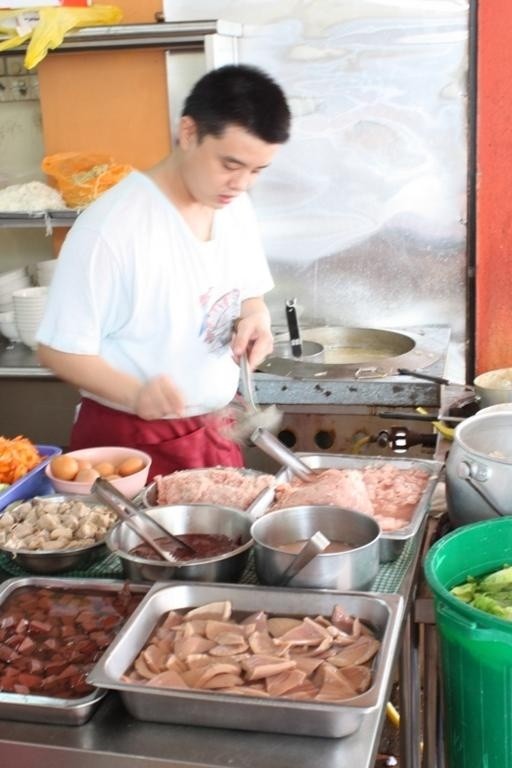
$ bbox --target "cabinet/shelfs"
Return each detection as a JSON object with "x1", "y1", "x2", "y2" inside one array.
[{"x1": 2, "y1": 202, "x2": 87, "y2": 380}]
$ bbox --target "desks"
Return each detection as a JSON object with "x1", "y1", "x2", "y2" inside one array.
[{"x1": 2, "y1": 478, "x2": 436, "y2": 768}]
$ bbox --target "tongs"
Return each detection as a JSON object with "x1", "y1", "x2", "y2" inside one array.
[
  {"x1": 87, "y1": 473, "x2": 198, "y2": 563},
  {"x1": 280, "y1": 530, "x2": 332, "y2": 591},
  {"x1": 247, "y1": 425, "x2": 314, "y2": 483}
]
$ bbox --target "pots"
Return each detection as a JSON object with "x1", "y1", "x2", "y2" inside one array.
[
  {"x1": 440, "y1": 407, "x2": 512, "y2": 527},
  {"x1": 395, "y1": 367, "x2": 512, "y2": 406}
]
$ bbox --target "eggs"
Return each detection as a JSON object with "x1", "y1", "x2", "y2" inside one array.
[{"x1": 50, "y1": 453, "x2": 143, "y2": 483}]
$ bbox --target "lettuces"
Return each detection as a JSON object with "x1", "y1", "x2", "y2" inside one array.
[{"x1": 449, "y1": 565, "x2": 512, "y2": 621}]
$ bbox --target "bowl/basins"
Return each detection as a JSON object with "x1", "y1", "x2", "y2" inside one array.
[
  {"x1": 1, "y1": 259, "x2": 58, "y2": 355},
  {"x1": 44, "y1": 444, "x2": 152, "y2": 505},
  {"x1": 2, "y1": 493, "x2": 128, "y2": 575},
  {"x1": 102, "y1": 506, "x2": 255, "y2": 582},
  {"x1": 144, "y1": 467, "x2": 269, "y2": 506},
  {"x1": 249, "y1": 505, "x2": 383, "y2": 594}
]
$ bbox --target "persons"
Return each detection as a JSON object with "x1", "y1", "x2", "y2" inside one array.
[{"x1": 32, "y1": 62, "x2": 296, "y2": 488}]
